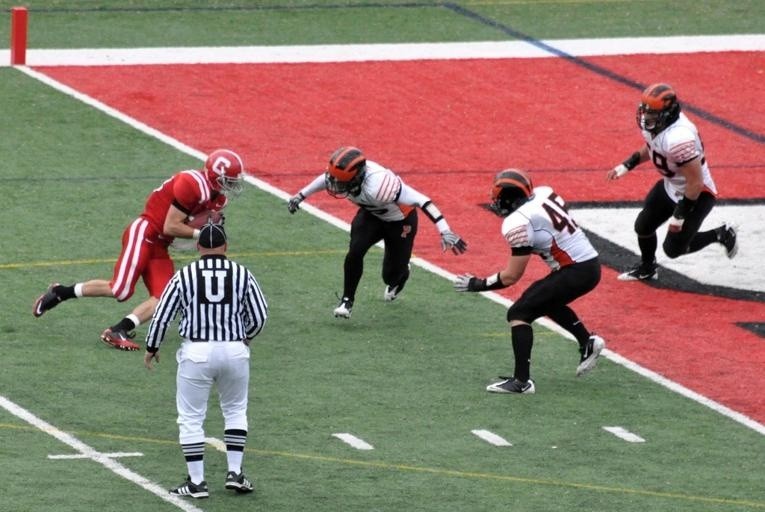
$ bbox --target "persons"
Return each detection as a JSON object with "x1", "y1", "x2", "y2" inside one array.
[
  {"x1": 143, "y1": 222, "x2": 268, "y2": 499},
  {"x1": 454, "y1": 168, "x2": 606, "y2": 393},
  {"x1": 33, "y1": 148, "x2": 244, "y2": 352},
  {"x1": 288, "y1": 146, "x2": 467, "y2": 319},
  {"x1": 607, "y1": 83, "x2": 740, "y2": 282}
]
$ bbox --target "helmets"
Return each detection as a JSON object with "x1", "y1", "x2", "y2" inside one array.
[
  {"x1": 203, "y1": 149, "x2": 244, "y2": 197},
  {"x1": 637, "y1": 83, "x2": 680, "y2": 131},
  {"x1": 323, "y1": 145, "x2": 366, "y2": 201},
  {"x1": 489, "y1": 167, "x2": 535, "y2": 214}
]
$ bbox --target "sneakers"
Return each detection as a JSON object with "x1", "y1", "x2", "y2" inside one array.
[
  {"x1": 100, "y1": 326, "x2": 140, "y2": 351},
  {"x1": 616, "y1": 264, "x2": 660, "y2": 282},
  {"x1": 574, "y1": 334, "x2": 605, "y2": 379},
  {"x1": 485, "y1": 374, "x2": 537, "y2": 395},
  {"x1": 31, "y1": 281, "x2": 61, "y2": 319},
  {"x1": 384, "y1": 263, "x2": 411, "y2": 301},
  {"x1": 224, "y1": 469, "x2": 255, "y2": 493},
  {"x1": 332, "y1": 297, "x2": 355, "y2": 319},
  {"x1": 724, "y1": 220, "x2": 739, "y2": 262},
  {"x1": 168, "y1": 480, "x2": 209, "y2": 498}
]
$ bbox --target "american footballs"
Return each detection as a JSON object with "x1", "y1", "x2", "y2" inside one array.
[{"x1": 186, "y1": 208, "x2": 221, "y2": 230}]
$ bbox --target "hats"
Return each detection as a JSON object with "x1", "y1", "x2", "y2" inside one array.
[{"x1": 198, "y1": 224, "x2": 227, "y2": 246}]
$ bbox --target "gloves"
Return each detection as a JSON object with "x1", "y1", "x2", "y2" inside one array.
[
  {"x1": 438, "y1": 228, "x2": 468, "y2": 256},
  {"x1": 476, "y1": 201, "x2": 501, "y2": 218},
  {"x1": 289, "y1": 194, "x2": 306, "y2": 215},
  {"x1": 194, "y1": 211, "x2": 225, "y2": 240}
]
